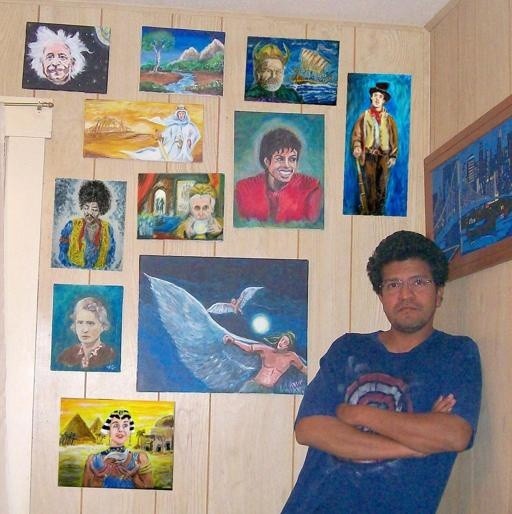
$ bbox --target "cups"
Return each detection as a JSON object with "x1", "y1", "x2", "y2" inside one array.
[{"x1": 378, "y1": 277, "x2": 433, "y2": 292}]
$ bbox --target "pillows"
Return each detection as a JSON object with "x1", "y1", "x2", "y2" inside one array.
[{"x1": 251, "y1": 41, "x2": 289, "y2": 65}]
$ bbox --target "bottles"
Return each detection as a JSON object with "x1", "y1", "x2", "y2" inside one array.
[{"x1": 369, "y1": 82, "x2": 391, "y2": 102}]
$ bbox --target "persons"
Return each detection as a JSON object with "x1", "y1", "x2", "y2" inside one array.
[
  {"x1": 82, "y1": 409, "x2": 153, "y2": 488},
  {"x1": 27, "y1": 25, "x2": 89, "y2": 85},
  {"x1": 58, "y1": 296, "x2": 116, "y2": 368},
  {"x1": 280, "y1": 231, "x2": 481, "y2": 514},
  {"x1": 223, "y1": 330, "x2": 307, "y2": 393},
  {"x1": 154, "y1": 105, "x2": 200, "y2": 163},
  {"x1": 235, "y1": 128, "x2": 321, "y2": 224},
  {"x1": 59, "y1": 181, "x2": 115, "y2": 270},
  {"x1": 226, "y1": 298, "x2": 242, "y2": 315},
  {"x1": 245, "y1": 41, "x2": 305, "y2": 104},
  {"x1": 176, "y1": 184, "x2": 224, "y2": 240},
  {"x1": 350, "y1": 82, "x2": 399, "y2": 215}
]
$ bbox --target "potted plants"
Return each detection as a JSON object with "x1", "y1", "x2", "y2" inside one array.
[{"x1": 421, "y1": 94, "x2": 512, "y2": 284}]
